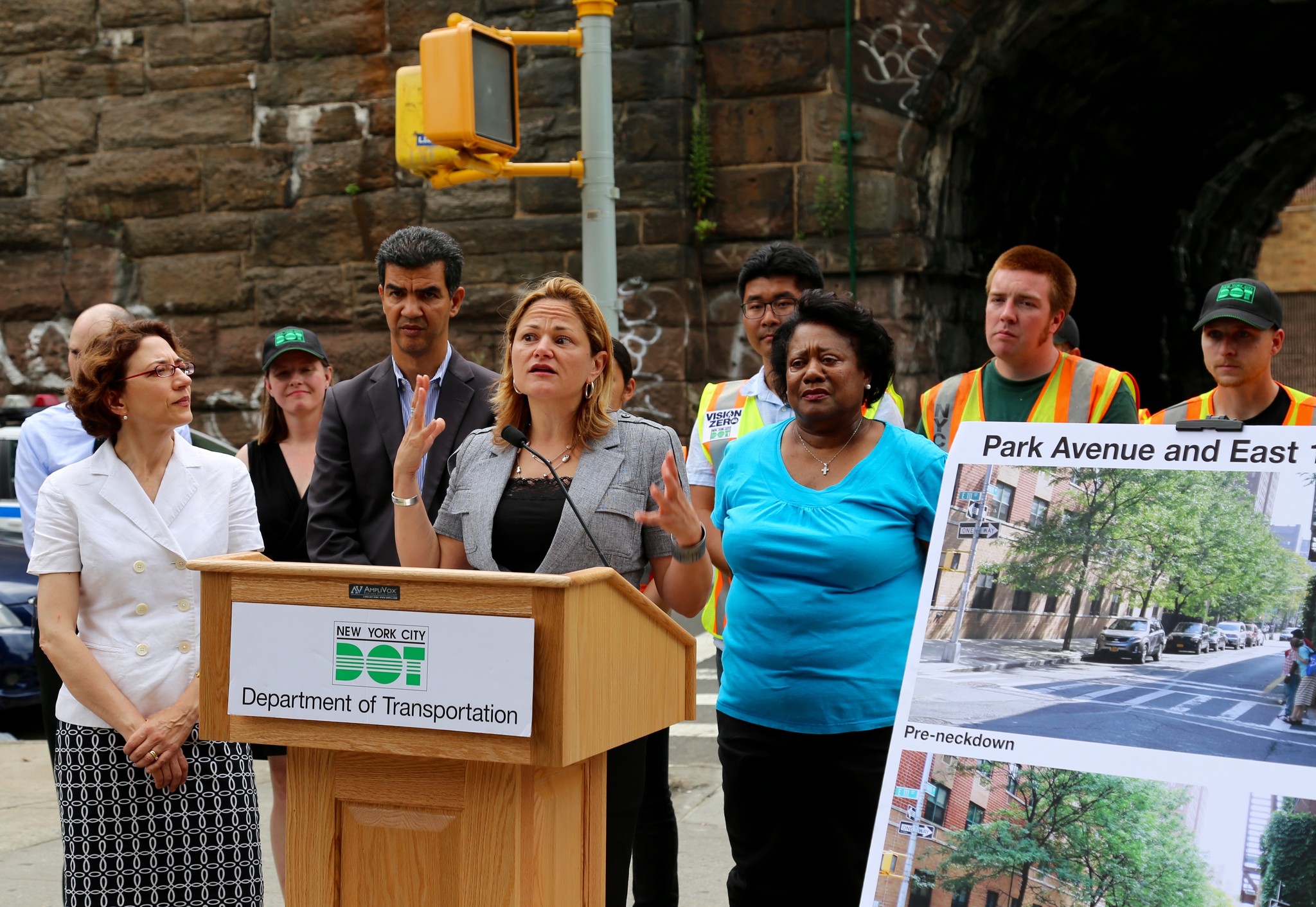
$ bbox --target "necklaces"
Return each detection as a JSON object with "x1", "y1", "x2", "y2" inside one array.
[
  {"x1": 515, "y1": 434, "x2": 578, "y2": 484},
  {"x1": 798, "y1": 419, "x2": 863, "y2": 475}
]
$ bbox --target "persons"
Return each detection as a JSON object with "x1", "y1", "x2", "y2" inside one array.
[
  {"x1": 232, "y1": 326, "x2": 334, "y2": 906},
  {"x1": 914, "y1": 244, "x2": 1148, "y2": 453},
  {"x1": 1147, "y1": 278, "x2": 1316, "y2": 426},
  {"x1": 709, "y1": 290, "x2": 950, "y2": 907},
  {"x1": 606, "y1": 337, "x2": 637, "y2": 415},
  {"x1": 14, "y1": 303, "x2": 193, "y2": 764},
  {"x1": 687, "y1": 244, "x2": 904, "y2": 685},
  {"x1": 27, "y1": 318, "x2": 265, "y2": 907},
  {"x1": 304, "y1": 226, "x2": 503, "y2": 568},
  {"x1": 392, "y1": 272, "x2": 713, "y2": 907},
  {"x1": 1278, "y1": 629, "x2": 1316, "y2": 724}
]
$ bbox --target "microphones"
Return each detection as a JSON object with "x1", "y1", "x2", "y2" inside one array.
[{"x1": 501, "y1": 424, "x2": 610, "y2": 568}]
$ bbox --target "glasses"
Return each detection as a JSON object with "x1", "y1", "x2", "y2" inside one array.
[
  {"x1": 115, "y1": 360, "x2": 195, "y2": 382},
  {"x1": 740, "y1": 296, "x2": 798, "y2": 320}
]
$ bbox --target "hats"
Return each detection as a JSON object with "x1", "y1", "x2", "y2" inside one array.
[
  {"x1": 1192, "y1": 278, "x2": 1283, "y2": 331},
  {"x1": 1291, "y1": 638, "x2": 1303, "y2": 645},
  {"x1": 1291, "y1": 630, "x2": 1304, "y2": 637},
  {"x1": 261, "y1": 326, "x2": 331, "y2": 371},
  {"x1": 1050, "y1": 314, "x2": 1079, "y2": 349}
]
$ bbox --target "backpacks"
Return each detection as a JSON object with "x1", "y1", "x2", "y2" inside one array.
[{"x1": 1303, "y1": 647, "x2": 1316, "y2": 676}]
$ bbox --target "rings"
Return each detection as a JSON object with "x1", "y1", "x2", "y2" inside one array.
[
  {"x1": 149, "y1": 750, "x2": 158, "y2": 760},
  {"x1": 411, "y1": 407, "x2": 416, "y2": 416}
]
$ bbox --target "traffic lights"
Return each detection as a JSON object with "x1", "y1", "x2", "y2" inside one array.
[
  {"x1": 419, "y1": 12, "x2": 520, "y2": 158},
  {"x1": 881, "y1": 850, "x2": 898, "y2": 875}
]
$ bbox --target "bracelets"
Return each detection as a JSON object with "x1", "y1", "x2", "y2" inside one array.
[
  {"x1": 392, "y1": 492, "x2": 419, "y2": 506},
  {"x1": 670, "y1": 522, "x2": 707, "y2": 563}
]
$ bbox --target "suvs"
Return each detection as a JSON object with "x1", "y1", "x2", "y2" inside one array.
[
  {"x1": 1245, "y1": 623, "x2": 1260, "y2": 648},
  {"x1": 1165, "y1": 621, "x2": 1211, "y2": 655},
  {"x1": 1094, "y1": 614, "x2": 1166, "y2": 664},
  {"x1": 1216, "y1": 620, "x2": 1247, "y2": 650}
]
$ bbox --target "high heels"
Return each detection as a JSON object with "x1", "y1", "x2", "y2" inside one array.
[
  {"x1": 1296, "y1": 719, "x2": 1302, "y2": 724},
  {"x1": 1282, "y1": 716, "x2": 1296, "y2": 725}
]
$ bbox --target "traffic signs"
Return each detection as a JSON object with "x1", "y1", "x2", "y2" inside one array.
[
  {"x1": 966, "y1": 498, "x2": 989, "y2": 522},
  {"x1": 898, "y1": 820, "x2": 935, "y2": 841},
  {"x1": 906, "y1": 804, "x2": 916, "y2": 822},
  {"x1": 957, "y1": 520, "x2": 1002, "y2": 539}
]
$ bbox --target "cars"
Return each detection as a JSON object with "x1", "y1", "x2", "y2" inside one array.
[
  {"x1": 1258, "y1": 627, "x2": 1265, "y2": 647},
  {"x1": 1208, "y1": 625, "x2": 1228, "y2": 652},
  {"x1": 1279, "y1": 627, "x2": 1299, "y2": 642},
  {"x1": 1, "y1": 390, "x2": 240, "y2": 741}
]
